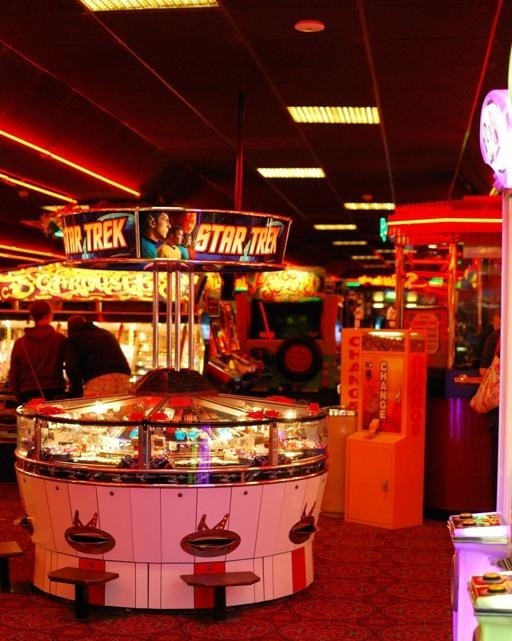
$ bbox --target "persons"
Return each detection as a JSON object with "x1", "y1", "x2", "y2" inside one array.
[
  {"x1": 9, "y1": 299, "x2": 132, "y2": 404},
  {"x1": 141, "y1": 211, "x2": 196, "y2": 259}
]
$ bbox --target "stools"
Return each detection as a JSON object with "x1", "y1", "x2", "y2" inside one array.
[
  {"x1": 182, "y1": 570, "x2": 260, "y2": 620},
  {"x1": 0, "y1": 540, "x2": 25, "y2": 592},
  {"x1": 46, "y1": 565, "x2": 119, "y2": 621}
]
